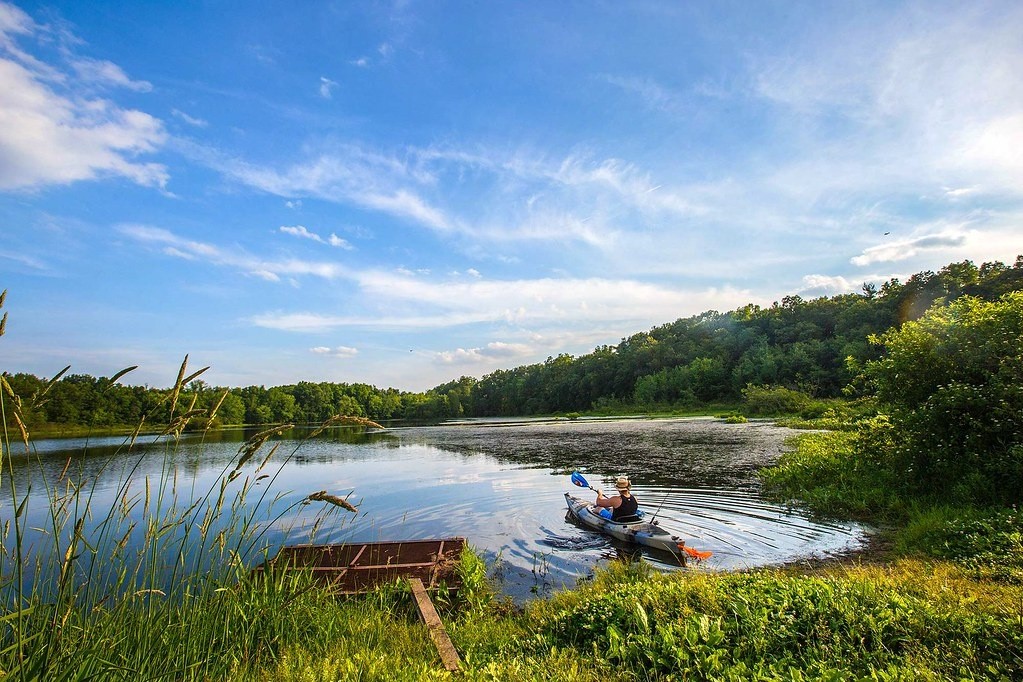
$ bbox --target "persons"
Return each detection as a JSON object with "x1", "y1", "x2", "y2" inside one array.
[{"x1": 593, "y1": 477, "x2": 638, "y2": 521}]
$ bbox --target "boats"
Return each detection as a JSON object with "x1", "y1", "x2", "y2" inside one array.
[{"x1": 564, "y1": 492, "x2": 685, "y2": 554}]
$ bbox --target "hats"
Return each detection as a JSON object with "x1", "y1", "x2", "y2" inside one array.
[{"x1": 614, "y1": 476, "x2": 631, "y2": 491}]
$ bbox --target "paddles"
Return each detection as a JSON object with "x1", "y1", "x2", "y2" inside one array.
[{"x1": 571, "y1": 471, "x2": 645, "y2": 518}]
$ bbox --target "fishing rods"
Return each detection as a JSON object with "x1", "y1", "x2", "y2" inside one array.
[{"x1": 650, "y1": 488, "x2": 673, "y2": 524}]
